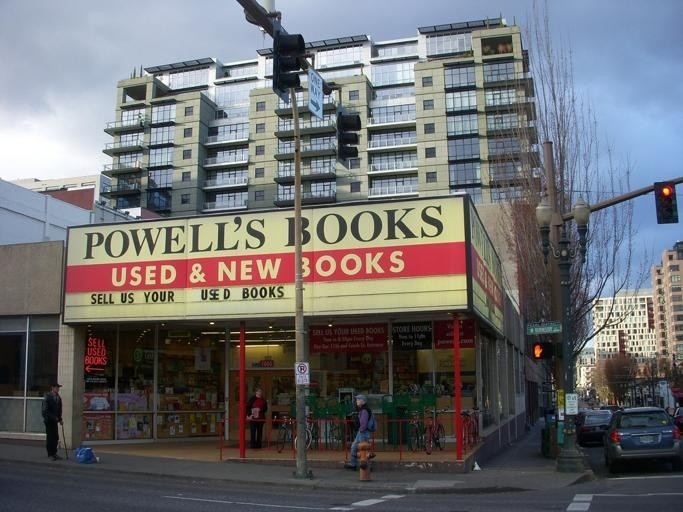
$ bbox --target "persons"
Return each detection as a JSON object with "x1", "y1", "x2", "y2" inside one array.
[
  {"x1": 343, "y1": 394, "x2": 376, "y2": 472},
  {"x1": 41, "y1": 382, "x2": 64, "y2": 460},
  {"x1": 245, "y1": 387, "x2": 267, "y2": 448}
]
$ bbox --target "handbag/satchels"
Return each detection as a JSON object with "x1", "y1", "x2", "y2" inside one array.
[{"x1": 75, "y1": 447, "x2": 104, "y2": 464}]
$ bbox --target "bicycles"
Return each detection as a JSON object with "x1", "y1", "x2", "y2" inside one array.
[{"x1": 274, "y1": 405, "x2": 481, "y2": 457}]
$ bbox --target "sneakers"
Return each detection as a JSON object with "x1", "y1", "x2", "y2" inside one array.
[{"x1": 47, "y1": 454, "x2": 61, "y2": 461}]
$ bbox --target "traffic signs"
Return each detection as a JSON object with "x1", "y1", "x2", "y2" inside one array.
[
  {"x1": 304, "y1": 62, "x2": 325, "y2": 120},
  {"x1": 524, "y1": 322, "x2": 562, "y2": 335}
]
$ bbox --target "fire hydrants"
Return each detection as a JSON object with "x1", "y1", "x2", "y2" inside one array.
[{"x1": 353, "y1": 439, "x2": 377, "y2": 482}]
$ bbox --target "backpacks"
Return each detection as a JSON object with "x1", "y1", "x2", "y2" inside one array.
[{"x1": 367, "y1": 413, "x2": 377, "y2": 432}]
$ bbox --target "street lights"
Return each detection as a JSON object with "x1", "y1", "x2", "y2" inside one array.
[{"x1": 531, "y1": 188, "x2": 594, "y2": 474}]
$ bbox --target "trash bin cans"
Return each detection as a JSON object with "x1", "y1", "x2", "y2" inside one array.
[{"x1": 541, "y1": 421, "x2": 576, "y2": 459}]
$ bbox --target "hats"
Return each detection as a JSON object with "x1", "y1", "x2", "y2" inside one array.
[
  {"x1": 51, "y1": 382, "x2": 63, "y2": 387},
  {"x1": 354, "y1": 394, "x2": 367, "y2": 401}
]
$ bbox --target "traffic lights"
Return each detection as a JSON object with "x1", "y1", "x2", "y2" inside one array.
[
  {"x1": 651, "y1": 178, "x2": 678, "y2": 224},
  {"x1": 333, "y1": 108, "x2": 362, "y2": 170},
  {"x1": 272, "y1": 30, "x2": 306, "y2": 105},
  {"x1": 531, "y1": 341, "x2": 553, "y2": 360}
]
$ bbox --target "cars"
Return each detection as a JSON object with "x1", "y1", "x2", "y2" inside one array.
[
  {"x1": 600, "y1": 406, "x2": 682, "y2": 474},
  {"x1": 574, "y1": 402, "x2": 682, "y2": 446}
]
